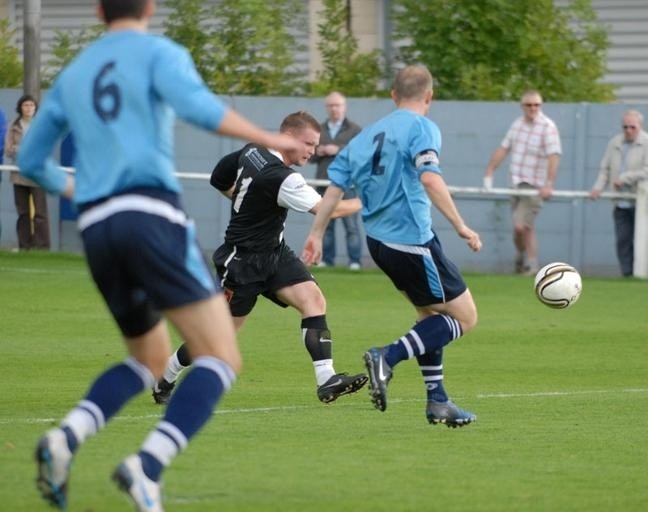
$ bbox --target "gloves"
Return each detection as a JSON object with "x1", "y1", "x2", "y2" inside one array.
[{"x1": 482, "y1": 176, "x2": 493, "y2": 193}]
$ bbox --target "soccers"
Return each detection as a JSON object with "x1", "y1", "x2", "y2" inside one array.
[{"x1": 535, "y1": 262, "x2": 581, "y2": 310}]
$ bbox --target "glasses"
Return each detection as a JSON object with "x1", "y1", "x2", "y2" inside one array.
[
  {"x1": 623, "y1": 125, "x2": 635, "y2": 129},
  {"x1": 525, "y1": 103, "x2": 541, "y2": 107}
]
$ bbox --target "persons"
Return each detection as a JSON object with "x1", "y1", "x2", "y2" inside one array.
[
  {"x1": 4, "y1": 94, "x2": 50, "y2": 254},
  {"x1": 483, "y1": 89, "x2": 561, "y2": 276},
  {"x1": 307, "y1": 91, "x2": 364, "y2": 273},
  {"x1": 301, "y1": 64, "x2": 481, "y2": 427},
  {"x1": 16, "y1": 4, "x2": 316, "y2": 509},
  {"x1": 590, "y1": 110, "x2": 648, "y2": 278},
  {"x1": 150, "y1": 113, "x2": 368, "y2": 405}
]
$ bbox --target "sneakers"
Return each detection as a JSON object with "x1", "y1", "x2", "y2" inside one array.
[
  {"x1": 426, "y1": 398, "x2": 477, "y2": 428},
  {"x1": 317, "y1": 262, "x2": 333, "y2": 270},
  {"x1": 512, "y1": 258, "x2": 538, "y2": 277},
  {"x1": 363, "y1": 347, "x2": 393, "y2": 412},
  {"x1": 349, "y1": 262, "x2": 361, "y2": 271},
  {"x1": 316, "y1": 371, "x2": 368, "y2": 405},
  {"x1": 152, "y1": 377, "x2": 176, "y2": 404},
  {"x1": 108, "y1": 453, "x2": 168, "y2": 511},
  {"x1": 34, "y1": 425, "x2": 73, "y2": 509}
]
